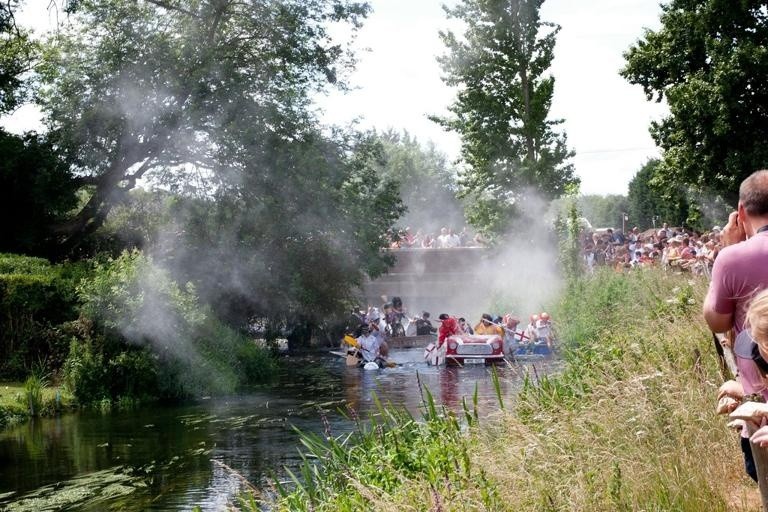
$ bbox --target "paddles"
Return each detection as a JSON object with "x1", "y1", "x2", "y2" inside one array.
[
  {"x1": 344, "y1": 336, "x2": 396, "y2": 368},
  {"x1": 424, "y1": 344, "x2": 442, "y2": 365},
  {"x1": 482, "y1": 318, "x2": 530, "y2": 342},
  {"x1": 346, "y1": 347, "x2": 359, "y2": 365}
]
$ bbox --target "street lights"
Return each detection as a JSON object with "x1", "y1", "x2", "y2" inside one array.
[{"x1": 622, "y1": 212, "x2": 629, "y2": 235}]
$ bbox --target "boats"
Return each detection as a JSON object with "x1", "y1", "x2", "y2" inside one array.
[
  {"x1": 448, "y1": 334, "x2": 505, "y2": 366},
  {"x1": 386, "y1": 336, "x2": 434, "y2": 349},
  {"x1": 511, "y1": 339, "x2": 560, "y2": 360}
]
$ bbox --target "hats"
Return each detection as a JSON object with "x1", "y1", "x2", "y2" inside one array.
[{"x1": 734, "y1": 329, "x2": 768, "y2": 373}]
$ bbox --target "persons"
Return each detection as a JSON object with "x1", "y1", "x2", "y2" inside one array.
[
  {"x1": 713, "y1": 371, "x2": 757, "y2": 485},
  {"x1": 700, "y1": 167, "x2": 767, "y2": 358},
  {"x1": 575, "y1": 210, "x2": 729, "y2": 279},
  {"x1": 728, "y1": 288, "x2": 768, "y2": 458},
  {"x1": 337, "y1": 294, "x2": 558, "y2": 370},
  {"x1": 376, "y1": 220, "x2": 491, "y2": 247}
]
